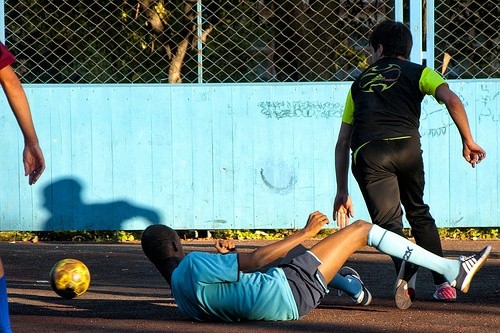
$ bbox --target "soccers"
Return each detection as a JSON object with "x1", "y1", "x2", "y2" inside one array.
[{"x1": 48, "y1": 257, "x2": 91, "y2": 299}]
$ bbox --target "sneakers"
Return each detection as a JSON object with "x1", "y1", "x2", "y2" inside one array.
[
  {"x1": 433, "y1": 284, "x2": 457, "y2": 301},
  {"x1": 450, "y1": 245, "x2": 492, "y2": 293},
  {"x1": 394, "y1": 260, "x2": 420, "y2": 310},
  {"x1": 338, "y1": 266, "x2": 372, "y2": 306}
]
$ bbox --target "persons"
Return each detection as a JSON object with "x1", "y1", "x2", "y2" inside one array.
[
  {"x1": 141, "y1": 211, "x2": 492, "y2": 323},
  {"x1": 332, "y1": 19, "x2": 489, "y2": 311},
  {"x1": 0, "y1": 40, "x2": 45, "y2": 333}
]
